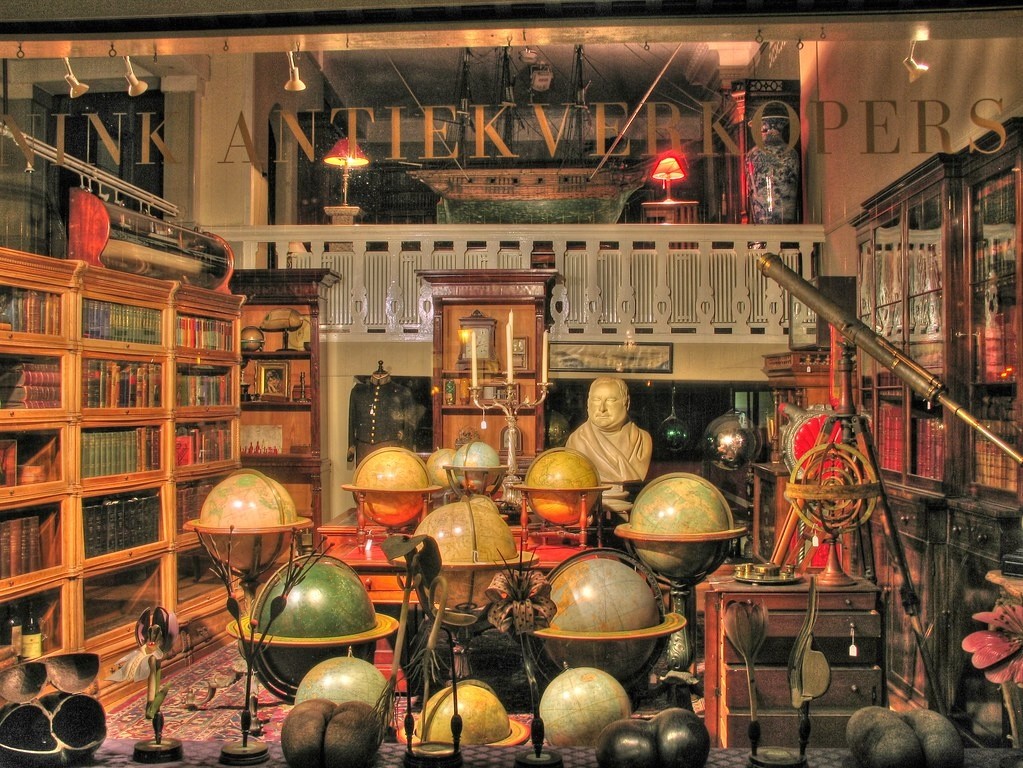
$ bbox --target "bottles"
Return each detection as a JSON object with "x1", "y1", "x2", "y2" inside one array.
[
  {"x1": 21, "y1": 595, "x2": 42, "y2": 661},
  {"x1": 2, "y1": 601, "x2": 21, "y2": 661}
]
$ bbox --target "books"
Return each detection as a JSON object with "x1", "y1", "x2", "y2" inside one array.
[
  {"x1": 81, "y1": 427, "x2": 162, "y2": 479},
  {"x1": 176, "y1": 315, "x2": 231, "y2": 351},
  {"x1": 0, "y1": 516, "x2": 42, "y2": 580},
  {"x1": 976, "y1": 238, "x2": 1016, "y2": 305},
  {"x1": 175, "y1": 429, "x2": 231, "y2": 466},
  {"x1": 176, "y1": 484, "x2": 215, "y2": 535},
  {"x1": 82, "y1": 298, "x2": 161, "y2": 345},
  {"x1": 82, "y1": 496, "x2": 162, "y2": 559},
  {"x1": 975, "y1": 420, "x2": 1019, "y2": 492},
  {"x1": 81, "y1": 358, "x2": 162, "y2": 408},
  {"x1": 974, "y1": 312, "x2": 1018, "y2": 383},
  {"x1": 973, "y1": 171, "x2": 1015, "y2": 240},
  {"x1": 0, "y1": 284, "x2": 62, "y2": 336},
  {"x1": 864, "y1": 400, "x2": 903, "y2": 473},
  {"x1": 0, "y1": 360, "x2": 61, "y2": 409},
  {"x1": 0, "y1": 644, "x2": 18, "y2": 671},
  {"x1": 916, "y1": 417, "x2": 945, "y2": 482},
  {"x1": 176, "y1": 373, "x2": 231, "y2": 407}
]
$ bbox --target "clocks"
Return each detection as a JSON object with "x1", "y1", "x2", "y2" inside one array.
[{"x1": 454, "y1": 309, "x2": 498, "y2": 372}]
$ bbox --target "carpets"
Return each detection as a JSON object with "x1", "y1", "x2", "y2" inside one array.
[{"x1": 105, "y1": 642, "x2": 537, "y2": 746}]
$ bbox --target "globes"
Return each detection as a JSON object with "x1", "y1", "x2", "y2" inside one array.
[
  {"x1": 182, "y1": 468, "x2": 314, "y2": 673},
  {"x1": 444, "y1": 441, "x2": 510, "y2": 501},
  {"x1": 523, "y1": 546, "x2": 689, "y2": 693},
  {"x1": 224, "y1": 552, "x2": 400, "y2": 704},
  {"x1": 293, "y1": 645, "x2": 395, "y2": 745},
  {"x1": 426, "y1": 448, "x2": 460, "y2": 507},
  {"x1": 507, "y1": 446, "x2": 612, "y2": 567},
  {"x1": 411, "y1": 495, "x2": 541, "y2": 690},
  {"x1": 538, "y1": 666, "x2": 632, "y2": 747},
  {"x1": 394, "y1": 681, "x2": 530, "y2": 749},
  {"x1": 241, "y1": 325, "x2": 265, "y2": 351},
  {"x1": 615, "y1": 471, "x2": 749, "y2": 709},
  {"x1": 341, "y1": 447, "x2": 443, "y2": 537}
]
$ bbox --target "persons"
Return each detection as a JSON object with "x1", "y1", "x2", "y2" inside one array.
[{"x1": 566, "y1": 374, "x2": 652, "y2": 498}]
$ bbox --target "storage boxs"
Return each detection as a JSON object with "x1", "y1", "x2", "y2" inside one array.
[{"x1": 789, "y1": 276, "x2": 855, "y2": 351}]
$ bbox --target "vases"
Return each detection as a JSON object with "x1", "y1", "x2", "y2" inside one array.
[{"x1": 747, "y1": 115, "x2": 799, "y2": 224}]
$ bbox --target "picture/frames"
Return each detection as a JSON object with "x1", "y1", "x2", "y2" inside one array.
[{"x1": 254, "y1": 359, "x2": 291, "y2": 401}]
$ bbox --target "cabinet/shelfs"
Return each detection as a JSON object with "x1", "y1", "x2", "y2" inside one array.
[
  {"x1": 697, "y1": 114, "x2": 1023, "y2": 750},
  {"x1": 298, "y1": 77, "x2": 803, "y2": 226},
  {"x1": 0, "y1": 246, "x2": 342, "y2": 710},
  {"x1": 414, "y1": 269, "x2": 559, "y2": 510}
]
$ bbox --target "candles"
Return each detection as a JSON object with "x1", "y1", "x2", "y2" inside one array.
[
  {"x1": 505, "y1": 322, "x2": 514, "y2": 382},
  {"x1": 509, "y1": 308, "x2": 513, "y2": 358},
  {"x1": 542, "y1": 330, "x2": 548, "y2": 383},
  {"x1": 471, "y1": 330, "x2": 478, "y2": 388}
]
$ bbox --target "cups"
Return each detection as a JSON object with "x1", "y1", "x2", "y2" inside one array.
[{"x1": 733, "y1": 562, "x2": 795, "y2": 580}]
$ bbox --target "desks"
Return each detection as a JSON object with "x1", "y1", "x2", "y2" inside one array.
[{"x1": 313, "y1": 508, "x2": 600, "y2": 604}]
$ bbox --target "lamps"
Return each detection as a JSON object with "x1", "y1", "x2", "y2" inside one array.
[
  {"x1": 530, "y1": 64, "x2": 553, "y2": 92},
  {"x1": 653, "y1": 158, "x2": 683, "y2": 202},
  {"x1": 123, "y1": 56, "x2": 148, "y2": 96},
  {"x1": 903, "y1": 40, "x2": 929, "y2": 84},
  {"x1": 62, "y1": 57, "x2": 89, "y2": 98},
  {"x1": 519, "y1": 49, "x2": 538, "y2": 63},
  {"x1": 284, "y1": 51, "x2": 306, "y2": 91},
  {"x1": 323, "y1": 138, "x2": 368, "y2": 207}
]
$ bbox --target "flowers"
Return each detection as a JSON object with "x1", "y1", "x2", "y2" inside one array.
[
  {"x1": 962, "y1": 604, "x2": 1023, "y2": 688},
  {"x1": 105, "y1": 606, "x2": 180, "y2": 746},
  {"x1": 485, "y1": 537, "x2": 556, "y2": 757}
]
